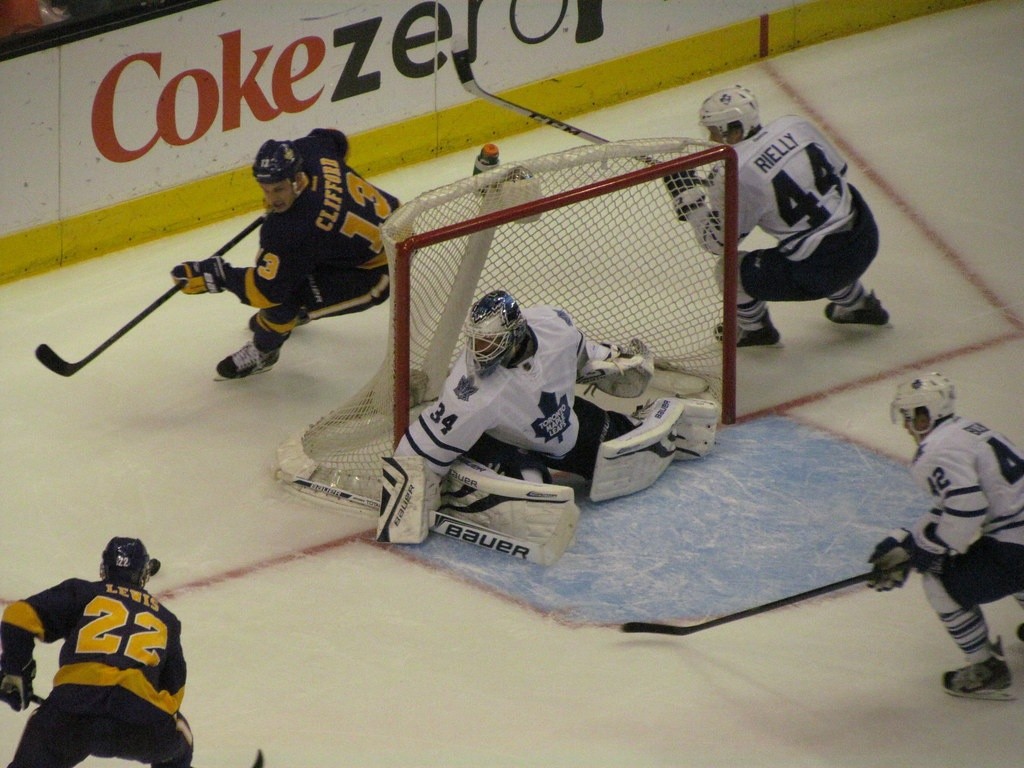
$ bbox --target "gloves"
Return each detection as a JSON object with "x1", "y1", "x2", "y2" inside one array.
[
  {"x1": 174, "y1": 256, "x2": 227, "y2": 295},
  {"x1": 0, "y1": 654, "x2": 36, "y2": 710},
  {"x1": 866, "y1": 528, "x2": 914, "y2": 591},
  {"x1": 664, "y1": 169, "x2": 706, "y2": 222}
]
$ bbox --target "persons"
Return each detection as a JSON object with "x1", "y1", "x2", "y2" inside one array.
[
  {"x1": 375, "y1": 290, "x2": 718, "y2": 546},
  {"x1": 0, "y1": 537, "x2": 194, "y2": 768},
  {"x1": 663, "y1": 85, "x2": 891, "y2": 348},
  {"x1": 865, "y1": 372, "x2": 1024, "y2": 699},
  {"x1": 170, "y1": 128, "x2": 416, "y2": 379}
]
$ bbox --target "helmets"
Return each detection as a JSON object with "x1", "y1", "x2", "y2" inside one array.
[
  {"x1": 894, "y1": 372, "x2": 956, "y2": 434},
  {"x1": 467, "y1": 291, "x2": 527, "y2": 378},
  {"x1": 101, "y1": 536, "x2": 152, "y2": 586},
  {"x1": 700, "y1": 87, "x2": 762, "y2": 144},
  {"x1": 252, "y1": 140, "x2": 305, "y2": 183}
]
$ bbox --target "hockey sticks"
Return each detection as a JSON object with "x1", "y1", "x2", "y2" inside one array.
[
  {"x1": 450, "y1": 33, "x2": 715, "y2": 188},
  {"x1": 620, "y1": 570, "x2": 880, "y2": 639},
  {"x1": 34, "y1": 206, "x2": 274, "y2": 379},
  {"x1": 30, "y1": 692, "x2": 265, "y2": 768}
]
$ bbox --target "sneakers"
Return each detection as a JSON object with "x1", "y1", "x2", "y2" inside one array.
[
  {"x1": 713, "y1": 309, "x2": 787, "y2": 349},
  {"x1": 942, "y1": 636, "x2": 1016, "y2": 699},
  {"x1": 824, "y1": 291, "x2": 893, "y2": 327},
  {"x1": 214, "y1": 334, "x2": 280, "y2": 382}
]
